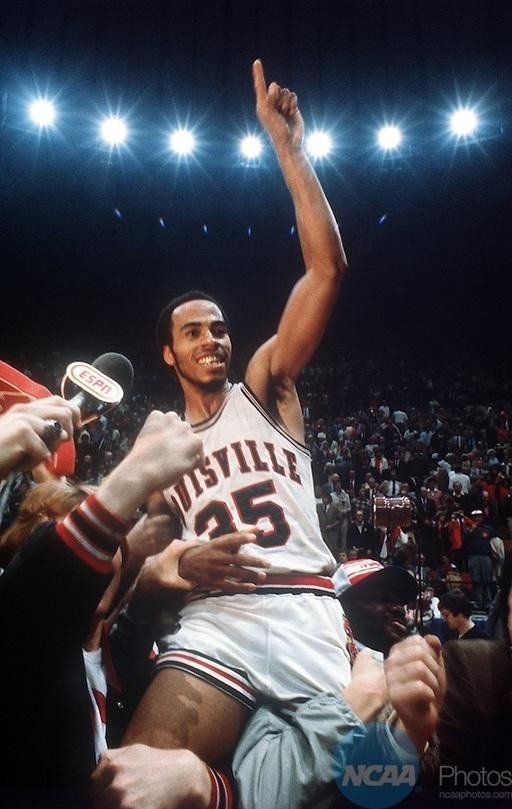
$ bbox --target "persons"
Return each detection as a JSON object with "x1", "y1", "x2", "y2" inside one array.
[
  {"x1": 0, "y1": 333, "x2": 510, "y2": 809},
  {"x1": 98, "y1": 56, "x2": 363, "y2": 770}
]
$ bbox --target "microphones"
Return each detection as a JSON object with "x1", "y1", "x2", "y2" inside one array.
[{"x1": 21, "y1": 352, "x2": 134, "y2": 470}]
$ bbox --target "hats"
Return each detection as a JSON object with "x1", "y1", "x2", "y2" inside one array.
[{"x1": 330, "y1": 557, "x2": 419, "y2": 605}]
